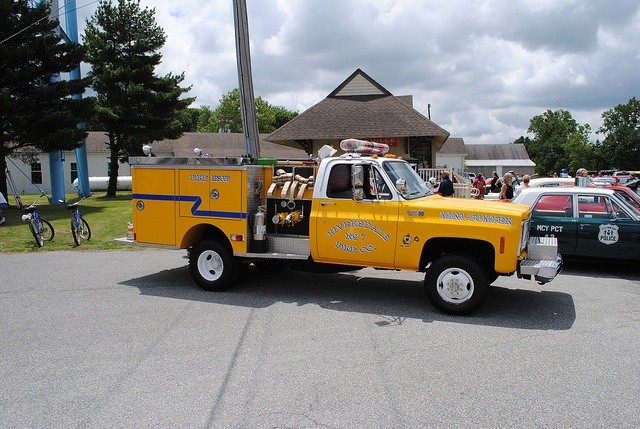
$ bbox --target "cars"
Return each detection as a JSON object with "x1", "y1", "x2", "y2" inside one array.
[
  {"x1": 508, "y1": 186, "x2": 640, "y2": 220},
  {"x1": 529, "y1": 187, "x2": 640, "y2": 260}
]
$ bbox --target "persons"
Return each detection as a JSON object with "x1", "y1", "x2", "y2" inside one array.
[
  {"x1": 472, "y1": 173, "x2": 486, "y2": 195},
  {"x1": 501, "y1": 173, "x2": 514, "y2": 199},
  {"x1": 516, "y1": 175, "x2": 531, "y2": 197},
  {"x1": 438, "y1": 171, "x2": 455, "y2": 198},
  {"x1": 490, "y1": 171, "x2": 501, "y2": 193}
]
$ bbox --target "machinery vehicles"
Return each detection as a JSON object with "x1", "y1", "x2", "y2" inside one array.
[{"x1": 129, "y1": 138, "x2": 564, "y2": 315}]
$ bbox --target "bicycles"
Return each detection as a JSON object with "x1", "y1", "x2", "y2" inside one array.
[
  {"x1": 19, "y1": 191, "x2": 54, "y2": 248},
  {"x1": 59, "y1": 194, "x2": 91, "y2": 245}
]
